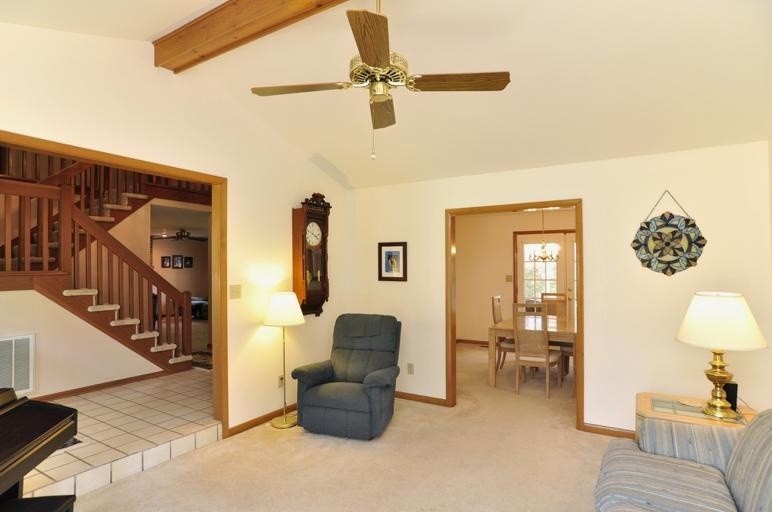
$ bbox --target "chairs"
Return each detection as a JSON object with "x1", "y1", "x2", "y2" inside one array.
[
  {"x1": 491, "y1": 293, "x2": 568, "y2": 401},
  {"x1": 291, "y1": 313, "x2": 401, "y2": 441}
]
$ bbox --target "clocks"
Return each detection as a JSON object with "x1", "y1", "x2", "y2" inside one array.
[{"x1": 293, "y1": 193, "x2": 332, "y2": 317}]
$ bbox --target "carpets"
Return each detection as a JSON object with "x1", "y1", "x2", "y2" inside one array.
[{"x1": 192, "y1": 351, "x2": 211, "y2": 370}]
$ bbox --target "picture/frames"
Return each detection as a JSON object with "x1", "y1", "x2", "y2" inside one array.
[
  {"x1": 378, "y1": 242, "x2": 408, "y2": 282},
  {"x1": 161, "y1": 255, "x2": 193, "y2": 269}
]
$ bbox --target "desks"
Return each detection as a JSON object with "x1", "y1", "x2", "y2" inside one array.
[{"x1": 488, "y1": 311, "x2": 576, "y2": 397}]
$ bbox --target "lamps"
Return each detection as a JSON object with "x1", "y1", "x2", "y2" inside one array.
[
  {"x1": 674, "y1": 291, "x2": 768, "y2": 419},
  {"x1": 529, "y1": 210, "x2": 560, "y2": 263},
  {"x1": 263, "y1": 291, "x2": 306, "y2": 429}
]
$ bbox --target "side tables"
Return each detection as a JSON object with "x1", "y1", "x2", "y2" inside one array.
[{"x1": 635, "y1": 392, "x2": 758, "y2": 441}]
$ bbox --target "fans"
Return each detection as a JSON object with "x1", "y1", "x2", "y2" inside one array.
[
  {"x1": 252, "y1": 0, "x2": 511, "y2": 129},
  {"x1": 152, "y1": 230, "x2": 208, "y2": 241}
]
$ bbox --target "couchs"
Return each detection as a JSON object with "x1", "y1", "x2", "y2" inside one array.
[{"x1": 594, "y1": 411, "x2": 771, "y2": 512}]
